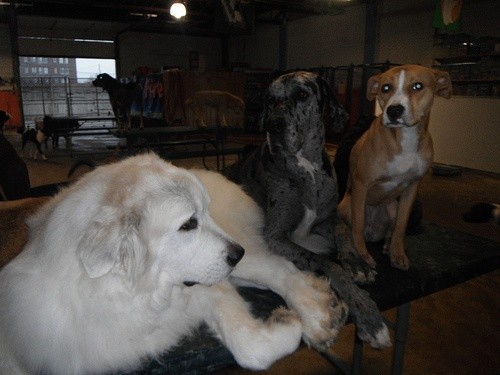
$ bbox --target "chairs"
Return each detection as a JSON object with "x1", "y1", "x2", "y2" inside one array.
[{"x1": 185, "y1": 91, "x2": 245, "y2": 173}]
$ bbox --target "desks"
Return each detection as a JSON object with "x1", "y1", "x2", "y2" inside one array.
[
  {"x1": 118, "y1": 150, "x2": 500, "y2": 375},
  {"x1": 108, "y1": 128, "x2": 231, "y2": 172}
]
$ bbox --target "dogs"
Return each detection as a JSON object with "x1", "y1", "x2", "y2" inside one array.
[
  {"x1": 0, "y1": 108, "x2": 32, "y2": 201},
  {"x1": 91, "y1": 72, "x2": 145, "y2": 129},
  {"x1": 212, "y1": 71, "x2": 393, "y2": 352},
  {"x1": 18, "y1": 111, "x2": 87, "y2": 160},
  {"x1": 338, "y1": 64, "x2": 455, "y2": 271},
  {"x1": 0, "y1": 152, "x2": 350, "y2": 375}
]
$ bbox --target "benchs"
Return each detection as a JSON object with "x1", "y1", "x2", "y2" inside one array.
[{"x1": 128, "y1": 141, "x2": 259, "y2": 172}]
